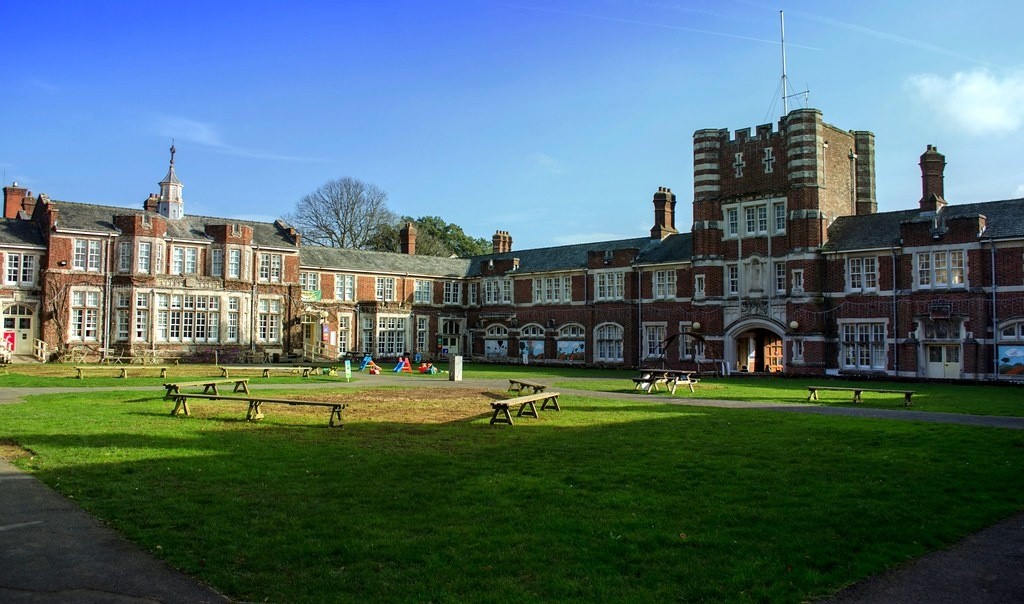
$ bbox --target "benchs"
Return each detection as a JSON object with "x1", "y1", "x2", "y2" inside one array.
[
  {"x1": 659, "y1": 376, "x2": 700, "y2": 393},
  {"x1": 169, "y1": 393, "x2": 349, "y2": 429},
  {"x1": 73, "y1": 366, "x2": 170, "y2": 379},
  {"x1": 490, "y1": 392, "x2": 561, "y2": 426},
  {"x1": 162, "y1": 378, "x2": 249, "y2": 401},
  {"x1": 218, "y1": 366, "x2": 312, "y2": 380},
  {"x1": 105, "y1": 356, "x2": 181, "y2": 365},
  {"x1": 508, "y1": 378, "x2": 545, "y2": 394},
  {"x1": 807, "y1": 385, "x2": 917, "y2": 407},
  {"x1": 632, "y1": 378, "x2": 673, "y2": 393}
]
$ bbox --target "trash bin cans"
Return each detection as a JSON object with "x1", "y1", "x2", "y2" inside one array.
[
  {"x1": 449, "y1": 355, "x2": 464, "y2": 381},
  {"x1": 273, "y1": 352, "x2": 280, "y2": 363}
]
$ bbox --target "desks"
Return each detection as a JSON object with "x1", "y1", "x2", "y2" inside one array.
[{"x1": 635, "y1": 369, "x2": 696, "y2": 394}]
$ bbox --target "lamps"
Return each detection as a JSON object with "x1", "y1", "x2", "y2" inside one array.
[
  {"x1": 61, "y1": 260, "x2": 67, "y2": 265},
  {"x1": 790, "y1": 321, "x2": 799, "y2": 330},
  {"x1": 693, "y1": 322, "x2": 700, "y2": 330}
]
